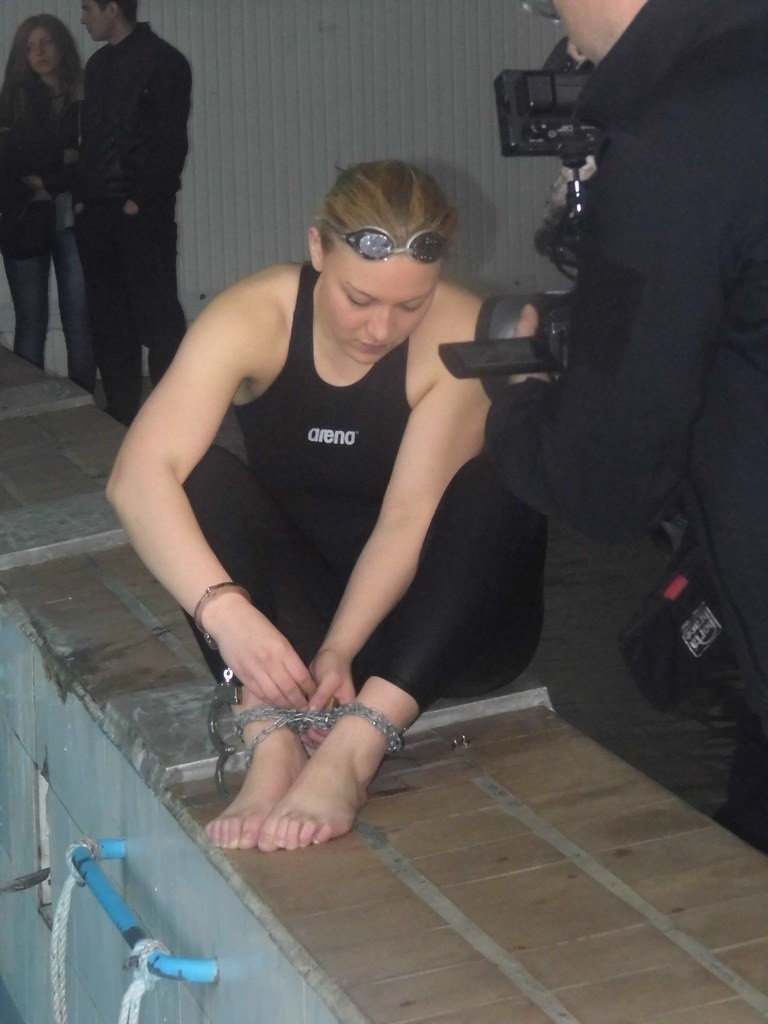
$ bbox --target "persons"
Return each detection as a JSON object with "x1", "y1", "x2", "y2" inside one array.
[
  {"x1": 490, "y1": 0, "x2": 768, "y2": 852},
  {"x1": 0, "y1": 0, "x2": 191, "y2": 421},
  {"x1": 104, "y1": 162, "x2": 547, "y2": 852}
]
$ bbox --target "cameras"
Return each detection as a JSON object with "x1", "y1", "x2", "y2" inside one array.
[{"x1": 440, "y1": 290, "x2": 572, "y2": 408}]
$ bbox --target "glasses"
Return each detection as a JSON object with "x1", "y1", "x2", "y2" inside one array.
[
  {"x1": 324, "y1": 219, "x2": 450, "y2": 263},
  {"x1": 521, "y1": 0, "x2": 561, "y2": 24}
]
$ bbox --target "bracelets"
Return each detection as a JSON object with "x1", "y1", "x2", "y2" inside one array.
[{"x1": 195, "y1": 582, "x2": 250, "y2": 648}]
